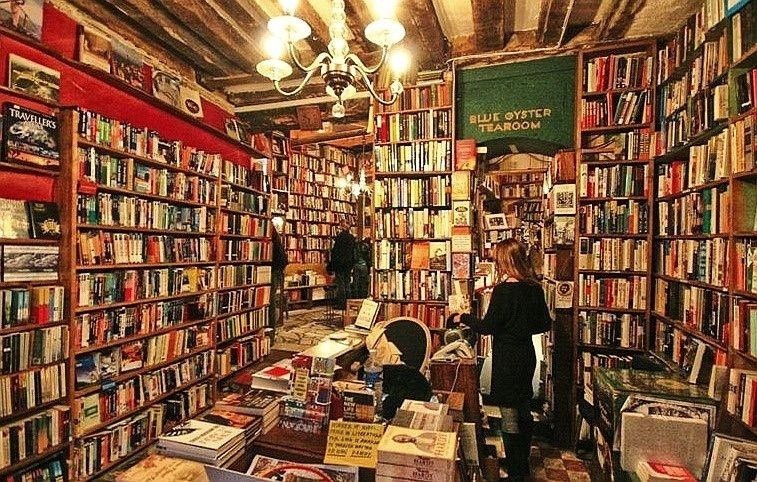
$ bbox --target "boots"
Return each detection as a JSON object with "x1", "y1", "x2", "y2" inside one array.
[
  {"x1": 500, "y1": 419, "x2": 533, "y2": 469},
  {"x1": 499, "y1": 432, "x2": 528, "y2": 482}
]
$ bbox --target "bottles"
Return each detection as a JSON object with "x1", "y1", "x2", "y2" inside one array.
[{"x1": 363, "y1": 348, "x2": 382, "y2": 388}]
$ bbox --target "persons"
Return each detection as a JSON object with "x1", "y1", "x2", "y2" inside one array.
[{"x1": 454, "y1": 238, "x2": 551, "y2": 480}]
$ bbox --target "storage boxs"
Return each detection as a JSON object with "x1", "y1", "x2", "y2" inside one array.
[
  {"x1": 591, "y1": 366, "x2": 718, "y2": 459},
  {"x1": 619, "y1": 393, "x2": 717, "y2": 474}
]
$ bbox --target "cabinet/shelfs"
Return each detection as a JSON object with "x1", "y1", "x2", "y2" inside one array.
[
  {"x1": 225, "y1": 121, "x2": 280, "y2": 388},
  {"x1": 0, "y1": 22, "x2": 68, "y2": 481},
  {"x1": 368, "y1": 73, "x2": 454, "y2": 333},
  {"x1": 573, "y1": 33, "x2": 661, "y2": 358},
  {"x1": 452, "y1": 46, "x2": 574, "y2": 353},
  {"x1": 277, "y1": 136, "x2": 370, "y2": 328},
  {"x1": 62, "y1": 63, "x2": 218, "y2": 480},
  {"x1": 648, "y1": 0, "x2": 757, "y2": 435}
]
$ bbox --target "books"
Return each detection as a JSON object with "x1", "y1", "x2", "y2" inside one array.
[
  {"x1": 158, "y1": 334, "x2": 483, "y2": 481},
  {"x1": 581, "y1": 0, "x2": 757, "y2": 482},
  {"x1": 371, "y1": 83, "x2": 473, "y2": 355},
  {"x1": 479, "y1": 333, "x2": 553, "y2": 398},
  {"x1": 1, "y1": 106, "x2": 358, "y2": 480},
  {"x1": 477, "y1": 152, "x2": 575, "y2": 309}
]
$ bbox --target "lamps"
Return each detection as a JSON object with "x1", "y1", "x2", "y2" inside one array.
[{"x1": 254, "y1": 0, "x2": 408, "y2": 118}]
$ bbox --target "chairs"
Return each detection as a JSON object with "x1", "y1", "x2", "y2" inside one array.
[{"x1": 381, "y1": 316, "x2": 432, "y2": 375}]
